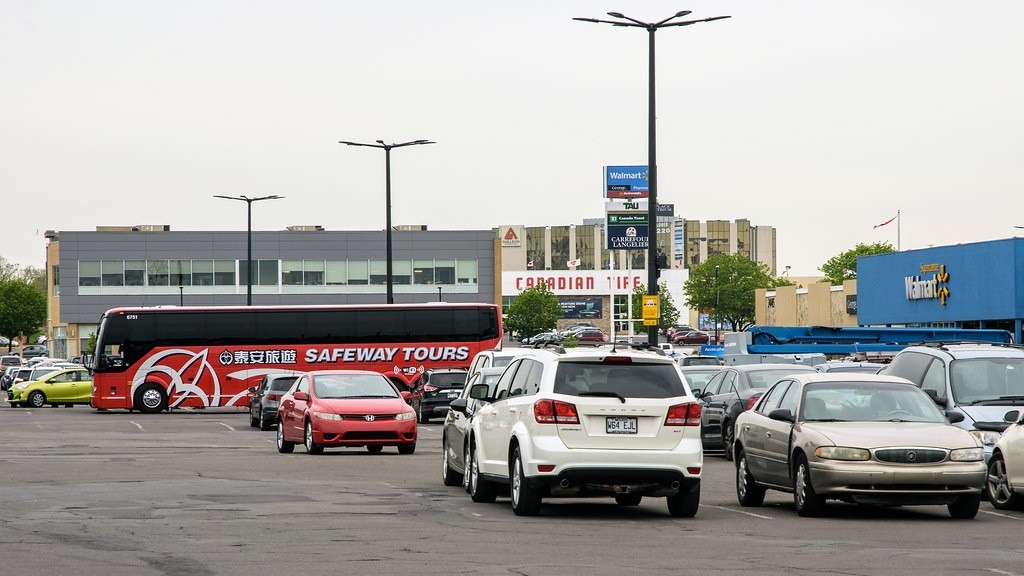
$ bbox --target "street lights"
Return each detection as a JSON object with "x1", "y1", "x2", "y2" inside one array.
[
  {"x1": 338, "y1": 140, "x2": 437, "y2": 303},
  {"x1": 212, "y1": 193, "x2": 286, "y2": 306},
  {"x1": 437, "y1": 287, "x2": 442, "y2": 302},
  {"x1": 689, "y1": 237, "x2": 728, "y2": 344},
  {"x1": 179, "y1": 287, "x2": 184, "y2": 306}
]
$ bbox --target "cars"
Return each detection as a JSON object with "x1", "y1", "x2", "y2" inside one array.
[
  {"x1": 659, "y1": 324, "x2": 711, "y2": 346},
  {"x1": 38, "y1": 335, "x2": 47, "y2": 345},
  {"x1": 673, "y1": 354, "x2": 887, "y2": 406},
  {"x1": 693, "y1": 364, "x2": 843, "y2": 459},
  {"x1": 443, "y1": 346, "x2": 531, "y2": 491},
  {"x1": 0, "y1": 336, "x2": 18, "y2": 347},
  {"x1": 733, "y1": 368, "x2": 988, "y2": 518},
  {"x1": 0, "y1": 345, "x2": 96, "y2": 408},
  {"x1": 276, "y1": 370, "x2": 418, "y2": 454},
  {"x1": 986, "y1": 407, "x2": 1024, "y2": 512},
  {"x1": 521, "y1": 320, "x2": 607, "y2": 345}
]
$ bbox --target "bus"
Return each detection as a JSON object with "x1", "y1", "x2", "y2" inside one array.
[{"x1": 82, "y1": 303, "x2": 502, "y2": 414}]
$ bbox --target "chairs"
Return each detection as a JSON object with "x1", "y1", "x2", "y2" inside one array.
[
  {"x1": 608, "y1": 368, "x2": 631, "y2": 388},
  {"x1": 871, "y1": 392, "x2": 896, "y2": 416},
  {"x1": 805, "y1": 397, "x2": 826, "y2": 418},
  {"x1": 686, "y1": 377, "x2": 706, "y2": 390},
  {"x1": 315, "y1": 381, "x2": 327, "y2": 394}
]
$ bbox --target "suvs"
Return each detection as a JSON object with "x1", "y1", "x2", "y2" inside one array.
[
  {"x1": 247, "y1": 373, "x2": 303, "y2": 430},
  {"x1": 883, "y1": 341, "x2": 1024, "y2": 462},
  {"x1": 409, "y1": 368, "x2": 468, "y2": 424},
  {"x1": 468, "y1": 346, "x2": 705, "y2": 516}
]
